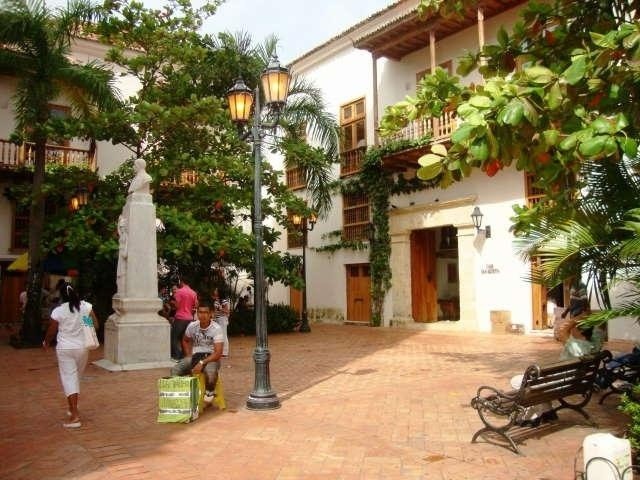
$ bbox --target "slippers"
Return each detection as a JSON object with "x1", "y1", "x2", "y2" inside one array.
[{"x1": 64, "y1": 410, "x2": 80, "y2": 428}]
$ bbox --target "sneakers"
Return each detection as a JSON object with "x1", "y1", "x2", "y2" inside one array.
[
  {"x1": 204, "y1": 389, "x2": 215, "y2": 402},
  {"x1": 514, "y1": 410, "x2": 557, "y2": 428}
]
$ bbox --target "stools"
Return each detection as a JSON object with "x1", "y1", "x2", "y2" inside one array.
[{"x1": 191, "y1": 369, "x2": 226, "y2": 414}]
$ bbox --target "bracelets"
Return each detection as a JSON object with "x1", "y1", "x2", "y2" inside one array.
[{"x1": 200, "y1": 360, "x2": 203, "y2": 365}]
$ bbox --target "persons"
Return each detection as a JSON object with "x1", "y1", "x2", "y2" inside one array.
[
  {"x1": 159, "y1": 275, "x2": 231, "y2": 363},
  {"x1": 511, "y1": 309, "x2": 607, "y2": 427},
  {"x1": 560, "y1": 275, "x2": 588, "y2": 318},
  {"x1": 19, "y1": 279, "x2": 67, "y2": 317},
  {"x1": 170, "y1": 303, "x2": 225, "y2": 407},
  {"x1": 43, "y1": 281, "x2": 100, "y2": 427}
]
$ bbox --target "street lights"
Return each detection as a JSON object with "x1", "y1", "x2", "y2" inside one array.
[
  {"x1": 225, "y1": 43, "x2": 292, "y2": 411},
  {"x1": 289, "y1": 207, "x2": 317, "y2": 333}
]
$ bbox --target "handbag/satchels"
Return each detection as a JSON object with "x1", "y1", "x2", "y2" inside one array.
[
  {"x1": 81, "y1": 316, "x2": 99, "y2": 350},
  {"x1": 193, "y1": 352, "x2": 211, "y2": 370}
]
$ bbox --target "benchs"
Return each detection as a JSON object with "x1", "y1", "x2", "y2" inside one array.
[{"x1": 470, "y1": 346, "x2": 640, "y2": 455}]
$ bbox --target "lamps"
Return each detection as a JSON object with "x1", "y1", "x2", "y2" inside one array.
[{"x1": 471, "y1": 207, "x2": 491, "y2": 240}]
